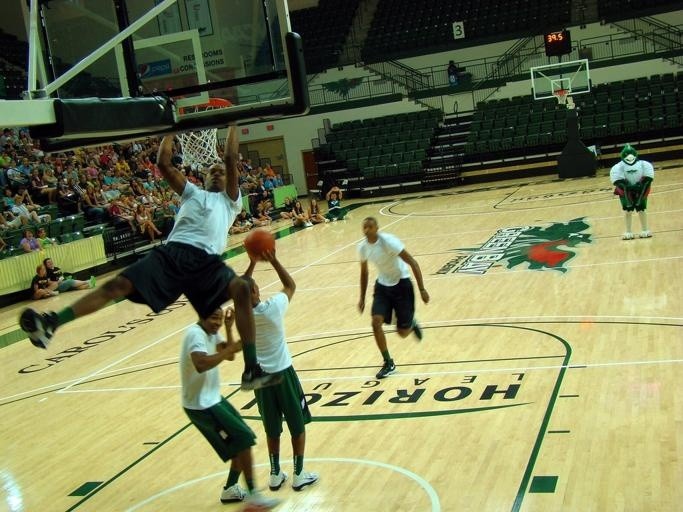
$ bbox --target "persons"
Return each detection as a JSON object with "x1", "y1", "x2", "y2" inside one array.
[
  {"x1": 356, "y1": 217, "x2": 431, "y2": 378},
  {"x1": 238, "y1": 248, "x2": 319, "y2": 491},
  {"x1": 447, "y1": 60, "x2": 457, "y2": 83},
  {"x1": 0, "y1": 58, "x2": 345, "y2": 298},
  {"x1": 179, "y1": 306, "x2": 281, "y2": 511},
  {"x1": 19, "y1": 122, "x2": 267, "y2": 391}
]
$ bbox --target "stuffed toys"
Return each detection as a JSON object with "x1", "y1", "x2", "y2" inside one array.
[{"x1": 609, "y1": 142, "x2": 655, "y2": 240}]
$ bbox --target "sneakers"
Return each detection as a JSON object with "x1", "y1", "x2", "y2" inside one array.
[
  {"x1": 305, "y1": 220, "x2": 313, "y2": 228},
  {"x1": 291, "y1": 470, "x2": 319, "y2": 492},
  {"x1": 19, "y1": 307, "x2": 58, "y2": 350},
  {"x1": 268, "y1": 469, "x2": 287, "y2": 491},
  {"x1": 89, "y1": 276, "x2": 97, "y2": 288},
  {"x1": 323, "y1": 215, "x2": 346, "y2": 223},
  {"x1": 220, "y1": 482, "x2": 247, "y2": 504},
  {"x1": 376, "y1": 359, "x2": 396, "y2": 379},
  {"x1": 413, "y1": 323, "x2": 422, "y2": 341},
  {"x1": 243, "y1": 490, "x2": 281, "y2": 511},
  {"x1": 240, "y1": 364, "x2": 259, "y2": 390}
]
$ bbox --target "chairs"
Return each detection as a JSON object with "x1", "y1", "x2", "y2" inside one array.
[{"x1": 0, "y1": 170, "x2": 169, "y2": 260}]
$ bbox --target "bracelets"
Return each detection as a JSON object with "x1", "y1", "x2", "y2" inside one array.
[{"x1": 419, "y1": 289, "x2": 424, "y2": 291}]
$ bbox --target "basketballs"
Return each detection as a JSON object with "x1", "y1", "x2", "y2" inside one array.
[{"x1": 244, "y1": 231, "x2": 275, "y2": 261}]
